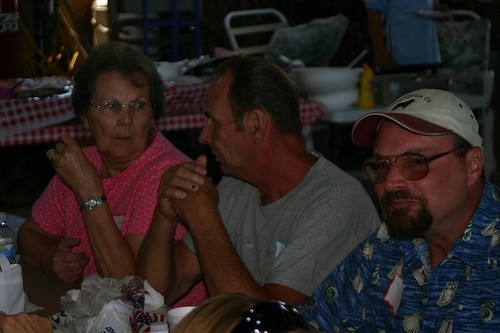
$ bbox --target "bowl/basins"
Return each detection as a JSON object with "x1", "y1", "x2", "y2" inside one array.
[
  {"x1": 155, "y1": 61, "x2": 185, "y2": 84},
  {"x1": 296, "y1": 67, "x2": 362, "y2": 93},
  {"x1": 371, "y1": 75, "x2": 455, "y2": 107}
]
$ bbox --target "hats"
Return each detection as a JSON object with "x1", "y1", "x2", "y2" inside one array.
[{"x1": 352, "y1": 89, "x2": 484, "y2": 156}]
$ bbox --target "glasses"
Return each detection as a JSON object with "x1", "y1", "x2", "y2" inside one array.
[
  {"x1": 90, "y1": 101, "x2": 155, "y2": 117},
  {"x1": 362, "y1": 143, "x2": 471, "y2": 185}
]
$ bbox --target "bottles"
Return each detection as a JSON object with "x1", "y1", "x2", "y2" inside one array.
[{"x1": 0, "y1": 212, "x2": 15, "y2": 263}]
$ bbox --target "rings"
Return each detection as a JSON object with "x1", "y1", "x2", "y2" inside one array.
[{"x1": 49, "y1": 152, "x2": 57, "y2": 160}]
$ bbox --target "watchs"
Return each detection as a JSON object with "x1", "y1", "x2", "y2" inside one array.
[{"x1": 80, "y1": 194, "x2": 106, "y2": 212}]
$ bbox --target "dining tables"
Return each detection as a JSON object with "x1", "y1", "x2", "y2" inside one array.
[{"x1": 0, "y1": 77, "x2": 491, "y2": 148}]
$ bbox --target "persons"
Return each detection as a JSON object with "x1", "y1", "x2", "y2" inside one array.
[
  {"x1": 365, "y1": 0, "x2": 442, "y2": 74},
  {"x1": 17, "y1": 41, "x2": 191, "y2": 283},
  {"x1": 175, "y1": 88, "x2": 500, "y2": 333},
  {"x1": 138, "y1": 57, "x2": 383, "y2": 308}
]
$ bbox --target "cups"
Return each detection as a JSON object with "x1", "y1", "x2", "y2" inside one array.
[{"x1": 168, "y1": 307, "x2": 199, "y2": 333}]
